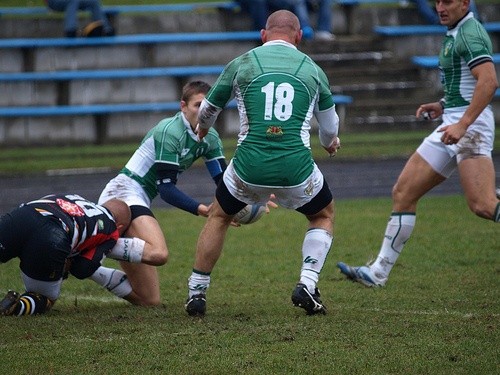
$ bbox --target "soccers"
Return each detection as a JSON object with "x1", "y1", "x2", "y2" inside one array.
[{"x1": 233, "y1": 204, "x2": 266, "y2": 224}]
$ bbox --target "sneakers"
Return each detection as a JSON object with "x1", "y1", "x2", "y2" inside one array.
[
  {"x1": 336, "y1": 261, "x2": 389, "y2": 288},
  {"x1": 183, "y1": 291, "x2": 208, "y2": 316},
  {"x1": 292, "y1": 283, "x2": 331, "y2": 317},
  {"x1": 0, "y1": 291, "x2": 21, "y2": 318}
]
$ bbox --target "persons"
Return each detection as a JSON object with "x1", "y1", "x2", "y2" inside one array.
[
  {"x1": 45, "y1": 0, "x2": 483, "y2": 47},
  {"x1": 336, "y1": 0, "x2": 500, "y2": 288},
  {"x1": 184, "y1": 9, "x2": 341, "y2": 316},
  {"x1": 0, "y1": 193, "x2": 131, "y2": 316},
  {"x1": 95, "y1": 81, "x2": 278, "y2": 306}
]
